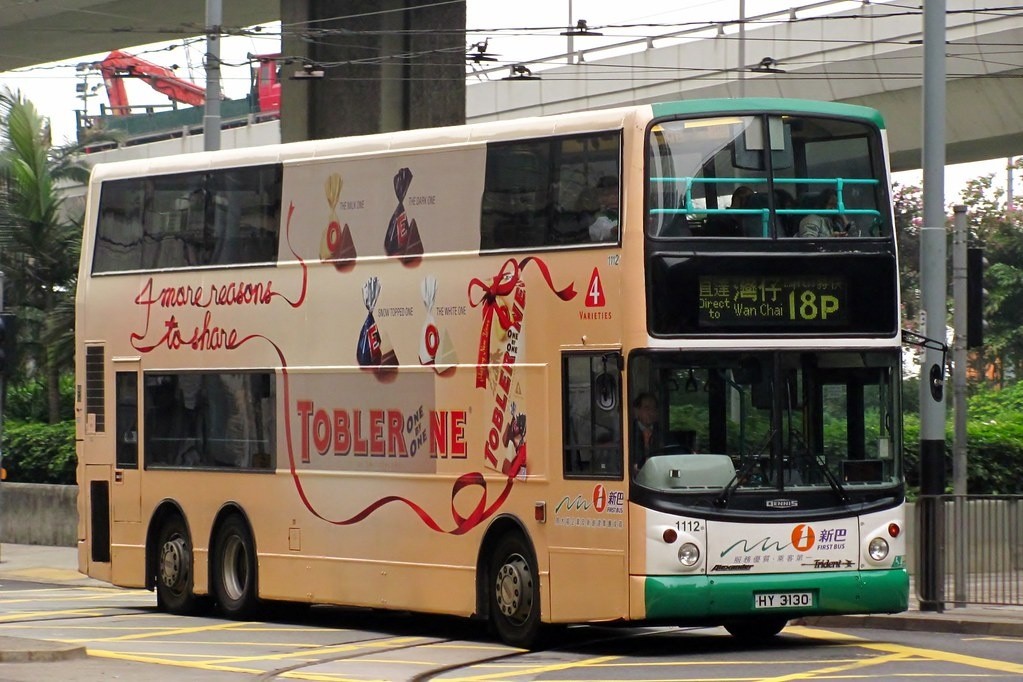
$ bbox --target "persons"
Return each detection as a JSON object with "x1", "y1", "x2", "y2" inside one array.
[
  {"x1": 798, "y1": 186, "x2": 858, "y2": 236},
  {"x1": 707, "y1": 186, "x2": 755, "y2": 237},
  {"x1": 634, "y1": 391, "x2": 660, "y2": 468},
  {"x1": 588, "y1": 175, "x2": 618, "y2": 242}
]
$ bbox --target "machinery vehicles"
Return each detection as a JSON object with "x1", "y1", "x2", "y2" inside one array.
[{"x1": 67, "y1": 42, "x2": 287, "y2": 154}]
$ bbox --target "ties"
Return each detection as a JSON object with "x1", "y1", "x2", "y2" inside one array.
[{"x1": 643, "y1": 428, "x2": 651, "y2": 449}]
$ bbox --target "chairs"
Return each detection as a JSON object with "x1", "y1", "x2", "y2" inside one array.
[
  {"x1": 794, "y1": 192, "x2": 820, "y2": 231},
  {"x1": 746, "y1": 193, "x2": 768, "y2": 237}
]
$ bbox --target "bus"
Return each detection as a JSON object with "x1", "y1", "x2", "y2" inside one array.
[{"x1": 69, "y1": 93, "x2": 950, "y2": 651}]
